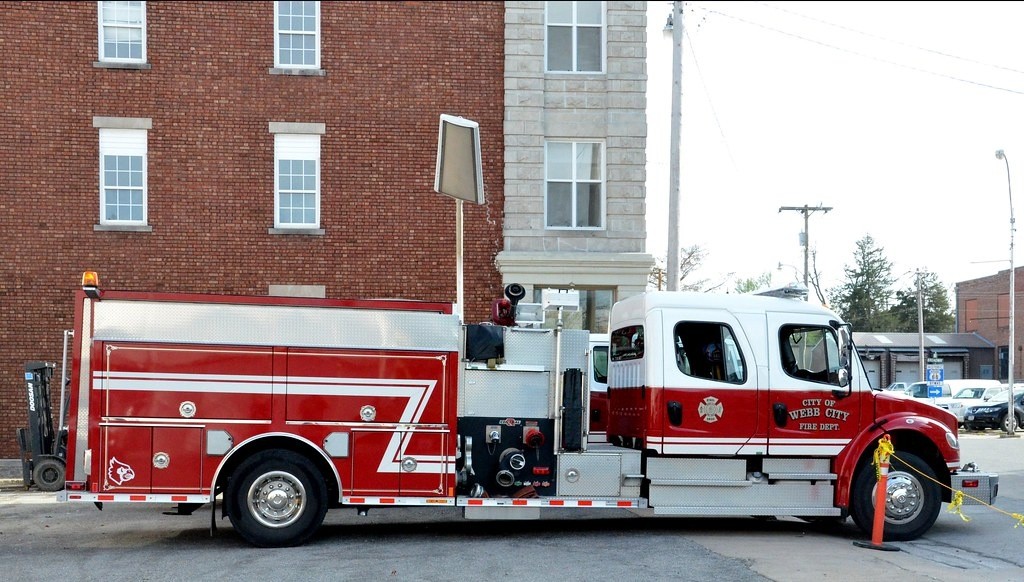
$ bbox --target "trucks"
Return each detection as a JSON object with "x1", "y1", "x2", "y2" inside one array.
[
  {"x1": 901, "y1": 379, "x2": 1001, "y2": 410},
  {"x1": 886, "y1": 382, "x2": 907, "y2": 394}
]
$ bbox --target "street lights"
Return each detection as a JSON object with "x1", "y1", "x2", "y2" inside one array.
[
  {"x1": 996, "y1": 150, "x2": 1016, "y2": 431},
  {"x1": 662, "y1": 15, "x2": 683, "y2": 293}
]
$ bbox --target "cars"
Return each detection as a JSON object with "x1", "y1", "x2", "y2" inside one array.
[
  {"x1": 961, "y1": 386, "x2": 1024, "y2": 432},
  {"x1": 934, "y1": 387, "x2": 1003, "y2": 429}
]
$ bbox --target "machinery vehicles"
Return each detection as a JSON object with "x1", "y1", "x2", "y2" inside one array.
[{"x1": 16, "y1": 362, "x2": 71, "y2": 490}]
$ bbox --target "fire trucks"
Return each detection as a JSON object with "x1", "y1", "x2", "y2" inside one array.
[{"x1": 59, "y1": 116, "x2": 1000, "y2": 549}]
